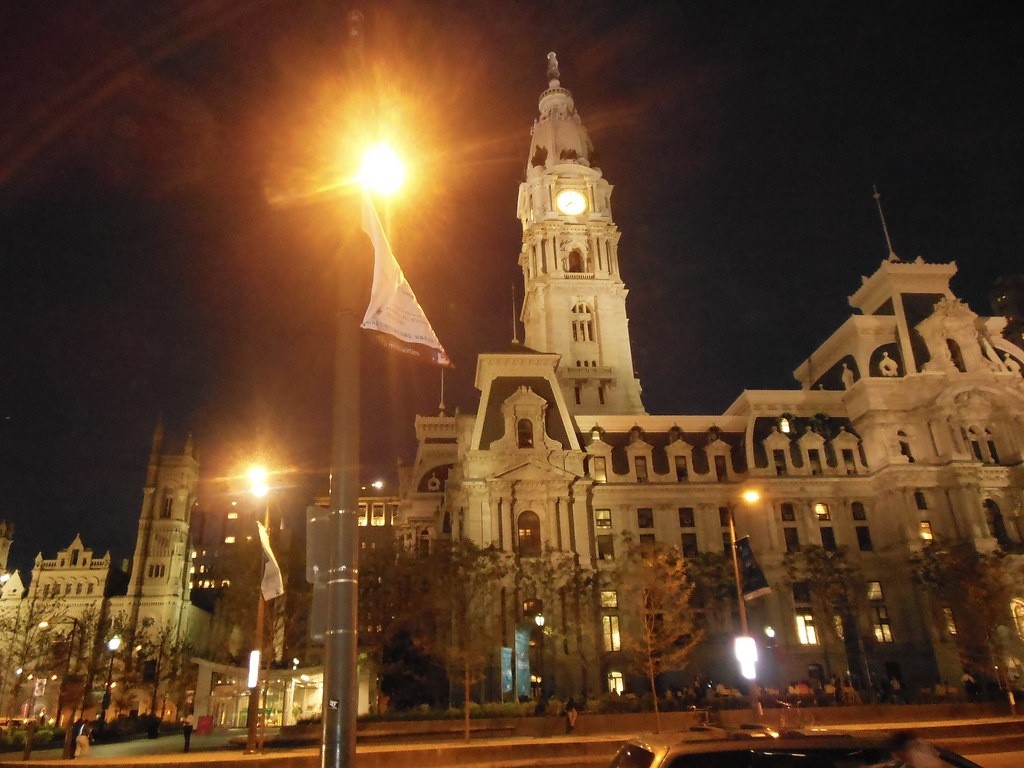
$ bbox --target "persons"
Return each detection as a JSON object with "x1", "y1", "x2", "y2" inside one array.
[
  {"x1": 69, "y1": 719, "x2": 92, "y2": 759},
  {"x1": 183, "y1": 721, "x2": 193, "y2": 752},
  {"x1": 535, "y1": 670, "x2": 976, "y2": 729}
]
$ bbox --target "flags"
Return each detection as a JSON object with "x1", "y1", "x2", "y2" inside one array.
[{"x1": 733, "y1": 538, "x2": 772, "y2": 599}]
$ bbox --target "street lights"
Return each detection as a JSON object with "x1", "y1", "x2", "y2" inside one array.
[
  {"x1": 763, "y1": 626, "x2": 784, "y2": 694},
  {"x1": 94, "y1": 635, "x2": 121, "y2": 740},
  {"x1": 726, "y1": 484, "x2": 762, "y2": 722},
  {"x1": 314, "y1": 143, "x2": 404, "y2": 768},
  {"x1": 534, "y1": 612, "x2": 546, "y2": 699}
]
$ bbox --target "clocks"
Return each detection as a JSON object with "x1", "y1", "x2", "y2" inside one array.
[{"x1": 556, "y1": 188, "x2": 589, "y2": 216}]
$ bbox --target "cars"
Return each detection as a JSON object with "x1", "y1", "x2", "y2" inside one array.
[{"x1": 602, "y1": 724, "x2": 987, "y2": 768}]
